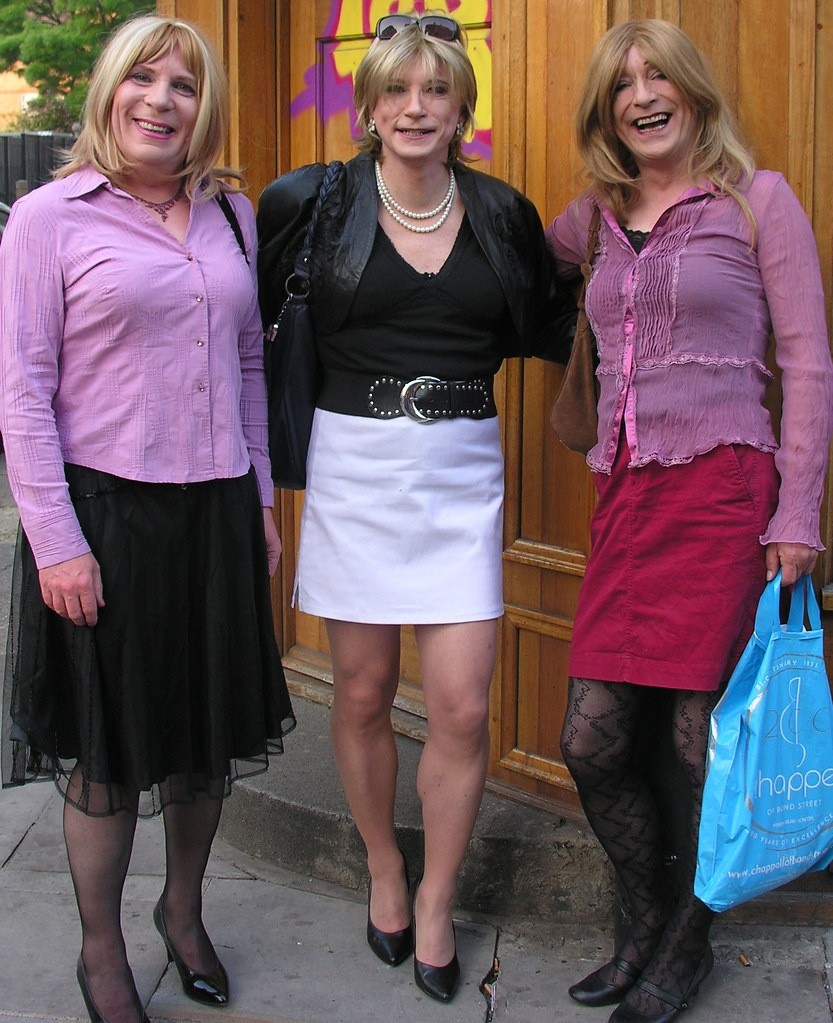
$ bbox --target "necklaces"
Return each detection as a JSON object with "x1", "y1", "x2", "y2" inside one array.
[
  {"x1": 374, "y1": 160, "x2": 455, "y2": 233},
  {"x1": 115, "y1": 181, "x2": 185, "y2": 223}
]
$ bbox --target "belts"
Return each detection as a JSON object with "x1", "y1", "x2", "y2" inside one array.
[{"x1": 313, "y1": 369, "x2": 498, "y2": 424}]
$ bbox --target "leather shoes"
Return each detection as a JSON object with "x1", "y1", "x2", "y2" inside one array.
[
  {"x1": 410, "y1": 874, "x2": 461, "y2": 1000},
  {"x1": 366, "y1": 872, "x2": 414, "y2": 967}
]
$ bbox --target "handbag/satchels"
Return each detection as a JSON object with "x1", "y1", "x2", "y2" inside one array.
[
  {"x1": 692, "y1": 570, "x2": 833, "y2": 911},
  {"x1": 259, "y1": 159, "x2": 348, "y2": 491}
]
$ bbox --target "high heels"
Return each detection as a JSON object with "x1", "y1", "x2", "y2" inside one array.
[
  {"x1": 153, "y1": 891, "x2": 229, "y2": 1006},
  {"x1": 77, "y1": 944, "x2": 151, "y2": 1023},
  {"x1": 607, "y1": 939, "x2": 714, "y2": 1023},
  {"x1": 568, "y1": 956, "x2": 642, "y2": 1007}
]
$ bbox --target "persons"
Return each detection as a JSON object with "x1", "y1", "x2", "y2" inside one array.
[
  {"x1": 543, "y1": 19, "x2": 833, "y2": 1023},
  {"x1": 256, "y1": 17, "x2": 596, "y2": 1002},
  {"x1": 0, "y1": 16, "x2": 282, "y2": 1023}
]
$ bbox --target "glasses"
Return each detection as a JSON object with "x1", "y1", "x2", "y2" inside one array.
[{"x1": 375, "y1": 14, "x2": 466, "y2": 48}]
